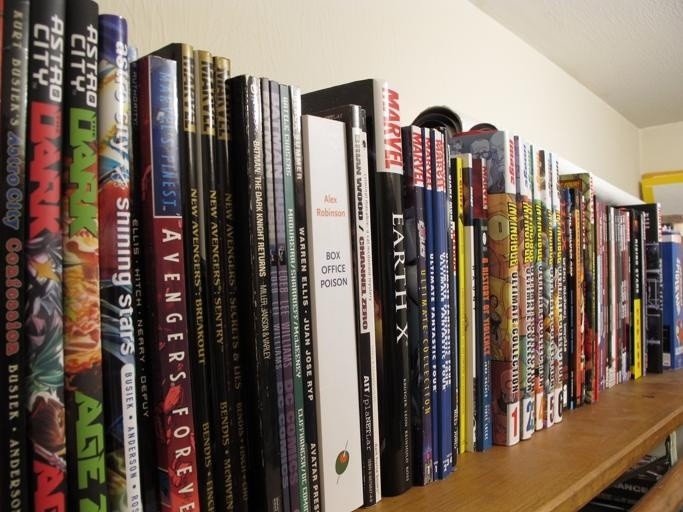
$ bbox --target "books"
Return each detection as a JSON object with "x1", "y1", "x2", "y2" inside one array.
[{"x1": 2, "y1": 0, "x2": 682, "y2": 512}]
